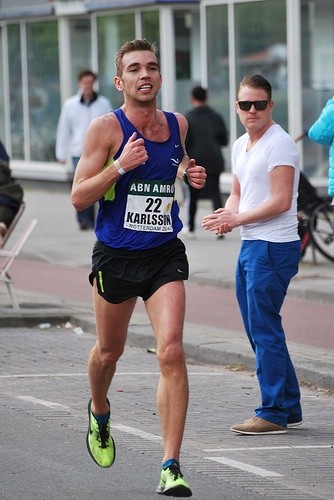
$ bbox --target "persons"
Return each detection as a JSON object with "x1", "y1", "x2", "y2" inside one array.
[
  {"x1": 55, "y1": 70, "x2": 115, "y2": 231},
  {"x1": 70, "y1": 39, "x2": 208, "y2": 496},
  {"x1": 202, "y1": 73, "x2": 304, "y2": 436},
  {"x1": 183, "y1": 86, "x2": 222, "y2": 238},
  {"x1": 0, "y1": 142, "x2": 24, "y2": 248},
  {"x1": 308, "y1": 97, "x2": 334, "y2": 195}
]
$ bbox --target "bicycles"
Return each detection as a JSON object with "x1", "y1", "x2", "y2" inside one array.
[{"x1": 297, "y1": 170, "x2": 334, "y2": 263}]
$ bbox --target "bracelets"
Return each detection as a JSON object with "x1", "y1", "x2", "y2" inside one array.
[{"x1": 182, "y1": 167, "x2": 189, "y2": 185}]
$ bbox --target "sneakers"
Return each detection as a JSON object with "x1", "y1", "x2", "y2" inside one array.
[
  {"x1": 86, "y1": 397, "x2": 116, "y2": 469},
  {"x1": 156, "y1": 461, "x2": 193, "y2": 497}
]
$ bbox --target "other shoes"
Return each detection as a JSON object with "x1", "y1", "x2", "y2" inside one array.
[
  {"x1": 230, "y1": 415, "x2": 287, "y2": 435},
  {"x1": 242, "y1": 417, "x2": 303, "y2": 427},
  {"x1": 215, "y1": 230, "x2": 225, "y2": 239},
  {"x1": 79, "y1": 220, "x2": 94, "y2": 229}
]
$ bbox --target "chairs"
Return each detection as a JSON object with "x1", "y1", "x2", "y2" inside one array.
[{"x1": 0, "y1": 200, "x2": 38, "y2": 309}]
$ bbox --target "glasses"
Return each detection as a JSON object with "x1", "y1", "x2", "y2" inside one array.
[{"x1": 238, "y1": 100, "x2": 269, "y2": 112}]
